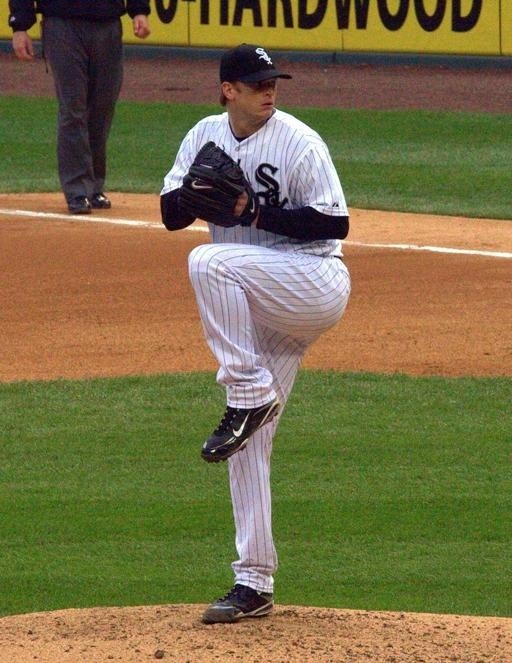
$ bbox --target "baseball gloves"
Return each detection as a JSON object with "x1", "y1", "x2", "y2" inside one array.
[{"x1": 174, "y1": 140, "x2": 260, "y2": 228}]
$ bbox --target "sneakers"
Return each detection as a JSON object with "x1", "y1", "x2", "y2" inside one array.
[
  {"x1": 200, "y1": 399, "x2": 281, "y2": 463},
  {"x1": 203, "y1": 585, "x2": 274, "y2": 623}
]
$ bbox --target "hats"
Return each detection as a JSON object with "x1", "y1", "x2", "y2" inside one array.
[{"x1": 220, "y1": 43, "x2": 292, "y2": 86}]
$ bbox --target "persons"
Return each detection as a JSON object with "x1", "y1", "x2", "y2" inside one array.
[
  {"x1": 157, "y1": 39, "x2": 355, "y2": 625},
  {"x1": 3, "y1": 0, "x2": 153, "y2": 214}
]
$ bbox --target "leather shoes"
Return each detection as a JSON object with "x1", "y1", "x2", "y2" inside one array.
[
  {"x1": 68, "y1": 196, "x2": 92, "y2": 214},
  {"x1": 91, "y1": 193, "x2": 111, "y2": 209}
]
